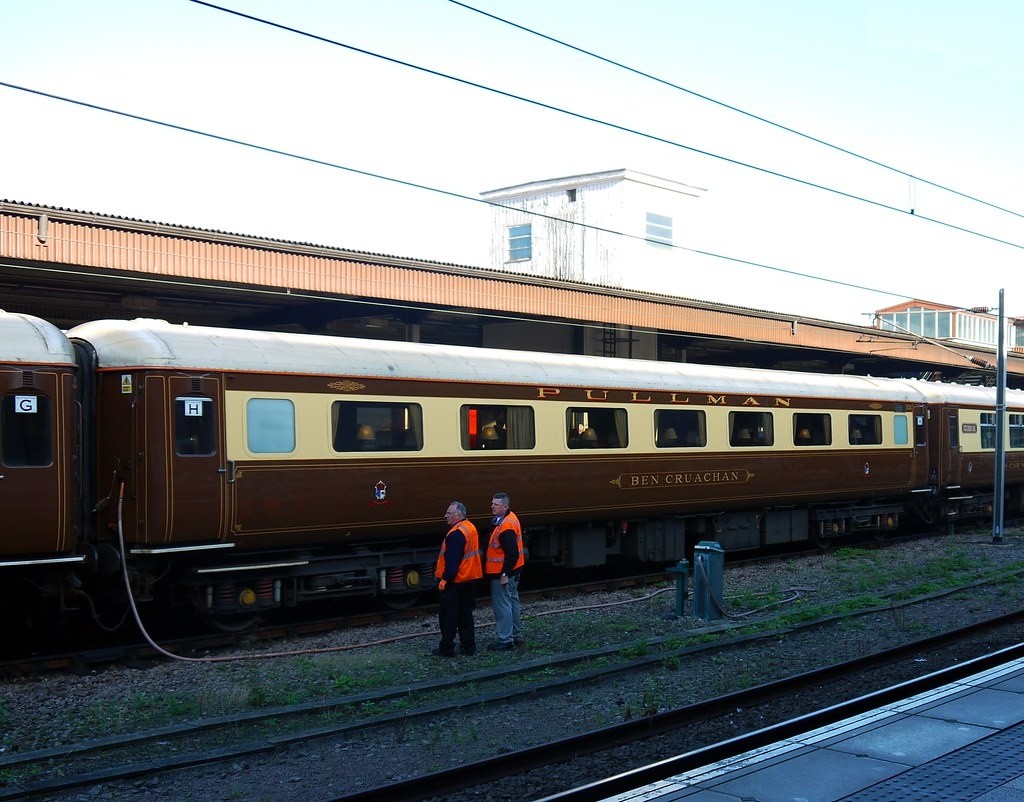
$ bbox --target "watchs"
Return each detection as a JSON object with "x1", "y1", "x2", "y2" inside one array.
[{"x1": 501, "y1": 572, "x2": 508, "y2": 577}]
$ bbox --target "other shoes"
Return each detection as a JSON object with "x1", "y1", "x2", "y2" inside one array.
[
  {"x1": 514, "y1": 637, "x2": 523, "y2": 645},
  {"x1": 488, "y1": 643, "x2": 513, "y2": 651},
  {"x1": 460, "y1": 644, "x2": 474, "y2": 656},
  {"x1": 432, "y1": 648, "x2": 455, "y2": 657}
]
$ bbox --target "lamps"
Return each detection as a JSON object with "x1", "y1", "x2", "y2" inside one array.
[
  {"x1": 479, "y1": 427, "x2": 499, "y2": 450},
  {"x1": 353, "y1": 424, "x2": 376, "y2": 451},
  {"x1": 583, "y1": 427, "x2": 598, "y2": 450},
  {"x1": 738, "y1": 429, "x2": 752, "y2": 446},
  {"x1": 851, "y1": 429, "x2": 863, "y2": 445},
  {"x1": 799, "y1": 429, "x2": 812, "y2": 445},
  {"x1": 662, "y1": 427, "x2": 678, "y2": 446}
]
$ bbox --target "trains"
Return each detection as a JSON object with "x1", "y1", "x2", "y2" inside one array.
[{"x1": 0, "y1": 309, "x2": 1024, "y2": 653}]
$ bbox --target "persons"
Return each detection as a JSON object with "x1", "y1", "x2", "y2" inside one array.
[
  {"x1": 484, "y1": 493, "x2": 523, "y2": 649},
  {"x1": 435, "y1": 501, "x2": 482, "y2": 657}
]
